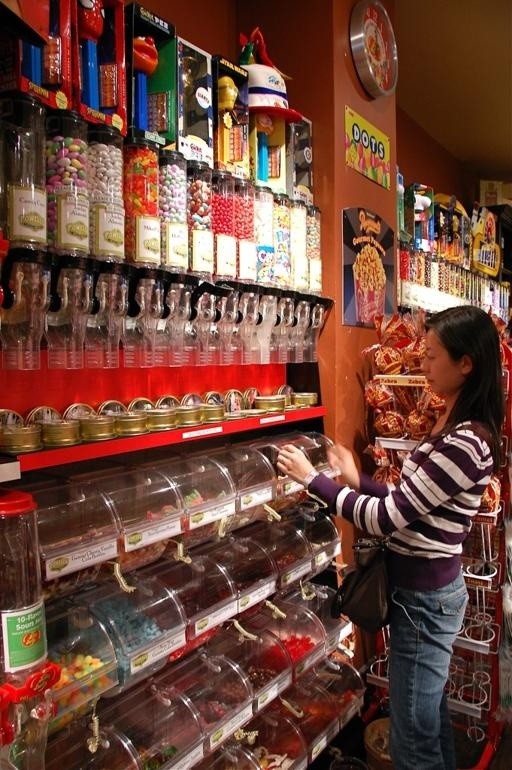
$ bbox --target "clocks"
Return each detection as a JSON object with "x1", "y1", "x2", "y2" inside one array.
[{"x1": 349, "y1": 0, "x2": 400, "y2": 99}]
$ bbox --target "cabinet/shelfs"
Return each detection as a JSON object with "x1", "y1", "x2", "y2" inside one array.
[
  {"x1": 2, "y1": 405, "x2": 369, "y2": 770},
  {"x1": 3, "y1": 67, "x2": 337, "y2": 310},
  {"x1": 397, "y1": 234, "x2": 512, "y2": 341},
  {"x1": 370, "y1": 372, "x2": 510, "y2": 770}
]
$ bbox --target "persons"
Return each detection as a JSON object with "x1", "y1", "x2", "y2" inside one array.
[{"x1": 272, "y1": 304, "x2": 504, "y2": 770}]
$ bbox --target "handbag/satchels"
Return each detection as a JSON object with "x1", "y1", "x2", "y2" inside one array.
[{"x1": 331, "y1": 535, "x2": 392, "y2": 634}]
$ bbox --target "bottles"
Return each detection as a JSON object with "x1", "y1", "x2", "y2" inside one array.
[{"x1": 1, "y1": 89, "x2": 323, "y2": 298}]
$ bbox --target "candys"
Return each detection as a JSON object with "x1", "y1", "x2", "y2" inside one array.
[
  {"x1": 399, "y1": 250, "x2": 471, "y2": 301},
  {"x1": 26, "y1": 462, "x2": 359, "y2": 770},
  {"x1": 9, "y1": 135, "x2": 322, "y2": 300}
]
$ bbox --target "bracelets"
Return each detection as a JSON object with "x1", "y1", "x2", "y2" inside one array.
[{"x1": 302, "y1": 470, "x2": 319, "y2": 488}]
